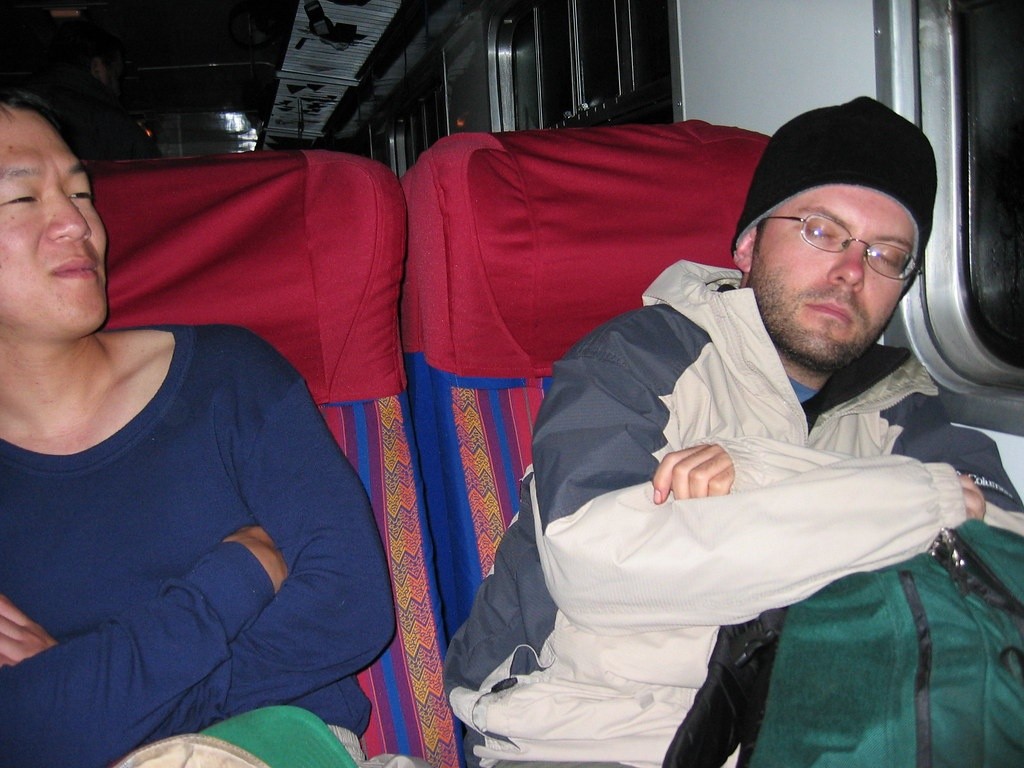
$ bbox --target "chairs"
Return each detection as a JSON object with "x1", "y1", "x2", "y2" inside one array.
[
  {"x1": 394, "y1": 118, "x2": 781, "y2": 724},
  {"x1": 55, "y1": 147, "x2": 468, "y2": 768}
]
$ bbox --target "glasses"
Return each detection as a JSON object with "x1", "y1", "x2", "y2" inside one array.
[{"x1": 764, "y1": 212, "x2": 922, "y2": 279}]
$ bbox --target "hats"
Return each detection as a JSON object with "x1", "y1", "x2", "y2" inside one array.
[{"x1": 729, "y1": 96, "x2": 940, "y2": 261}]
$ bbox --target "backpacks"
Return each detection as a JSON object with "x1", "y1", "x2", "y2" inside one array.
[{"x1": 659, "y1": 518, "x2": 1024, "y2": 768}]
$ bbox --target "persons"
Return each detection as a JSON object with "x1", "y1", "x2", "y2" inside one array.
[
  {"x1": 0, "y1": 87, "x2": 430, "y2": 767},
  {"x1": 443, "y1": 95, "x2": 1024, "y2": 768}
]
